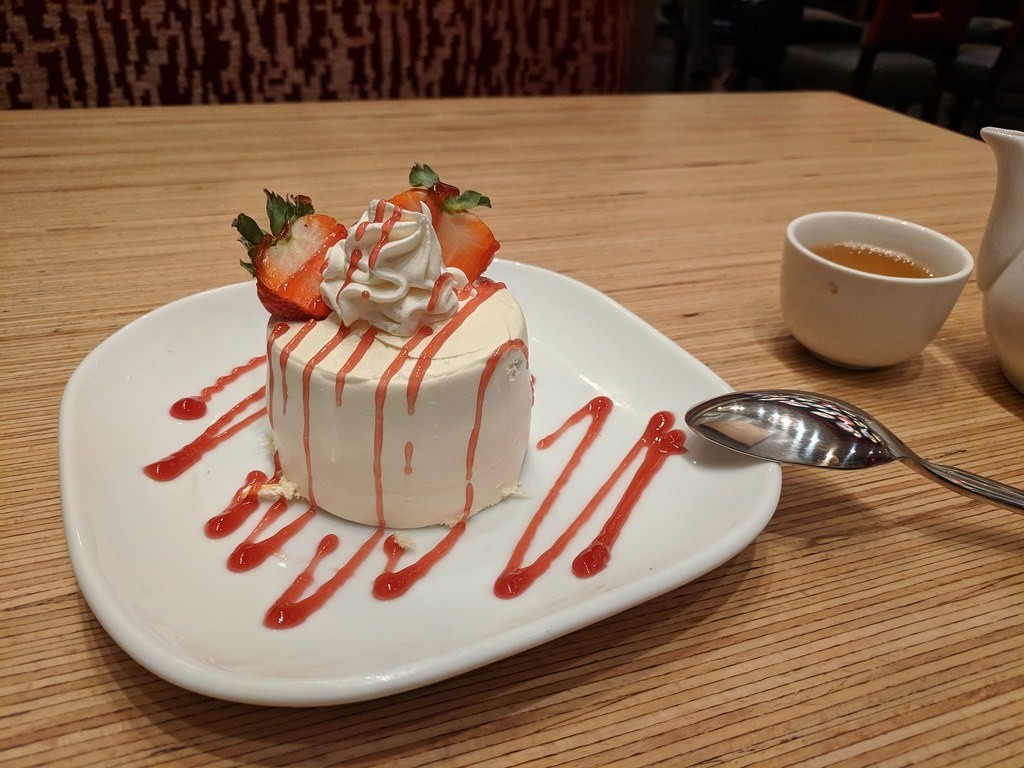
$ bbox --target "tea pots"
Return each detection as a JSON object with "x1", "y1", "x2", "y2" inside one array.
[{"x1": 974, "y1": 121, "x2": 1024, "y2": 401}]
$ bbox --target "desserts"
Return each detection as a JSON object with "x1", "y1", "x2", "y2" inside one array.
[{"x1": 229, "y1": 164, "x2": 536, "y2": 529}]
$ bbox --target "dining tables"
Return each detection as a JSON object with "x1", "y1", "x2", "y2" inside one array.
[{"x1": 0, "y1": 78, "x2": 1024, "y2": 768}]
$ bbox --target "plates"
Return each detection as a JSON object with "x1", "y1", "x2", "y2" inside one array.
[{"x1": 56, "y1": 247, "x2": 785, "y2": 710}]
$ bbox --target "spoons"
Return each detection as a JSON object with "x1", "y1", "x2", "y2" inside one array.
[{"x1": 686, "y1": 387, "x2": 1024, "y2": 516}]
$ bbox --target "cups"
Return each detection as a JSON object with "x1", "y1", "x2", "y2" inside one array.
[{"x1": 777, "y1": 207, "x2": 977, "y2": 373}]
